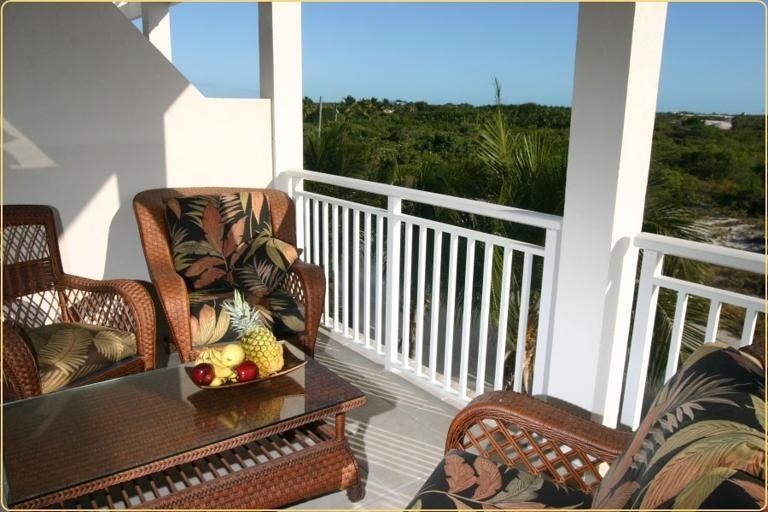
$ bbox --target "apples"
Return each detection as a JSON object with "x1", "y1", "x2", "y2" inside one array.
[
  {"x1": 232, "y1": 360, "x2": 257, "y2": 381},
  {"x1": 220, "y1": 344, "x2": 248, "y2": 365},
  {"x1": 191, "y1": 363, "x2": 214, "y2": 384}
]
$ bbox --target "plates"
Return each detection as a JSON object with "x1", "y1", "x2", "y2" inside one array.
[
  {"x1": 186, "y1": 374, "x2": 306, "y2": 418},
  {"x1": 180, "y1": 340, "x2": 313, "y2": 389}
]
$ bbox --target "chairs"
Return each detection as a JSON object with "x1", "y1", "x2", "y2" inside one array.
[
  {"x1": 1, "y1": 205, "x2": 157, "y2": 401},
  {"x1": 404, "y1": 341, "x2": 766, "y2": 511},
  {"x1": 132, "y1": 187, "x2": 326, "y2": 362}
]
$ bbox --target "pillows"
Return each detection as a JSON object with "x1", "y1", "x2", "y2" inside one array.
[
  {"x1": 223, "y1": 231, "x2": 304, "y2": 296},
  {"x1": 163, "y1": 191, "x2": 272, "y2": 292},
  {"x1": 590, "y1": 341, "x2": 767, "y2": 509}
]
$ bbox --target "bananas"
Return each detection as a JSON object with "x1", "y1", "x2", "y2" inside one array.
[{"x1": 195, "y1": 348, "x2": 238, "y2": 386}]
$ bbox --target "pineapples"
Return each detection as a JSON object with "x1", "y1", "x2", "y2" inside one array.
[{"x1": 218, "y1": 287, "x2": 284, "y2": 376}]
